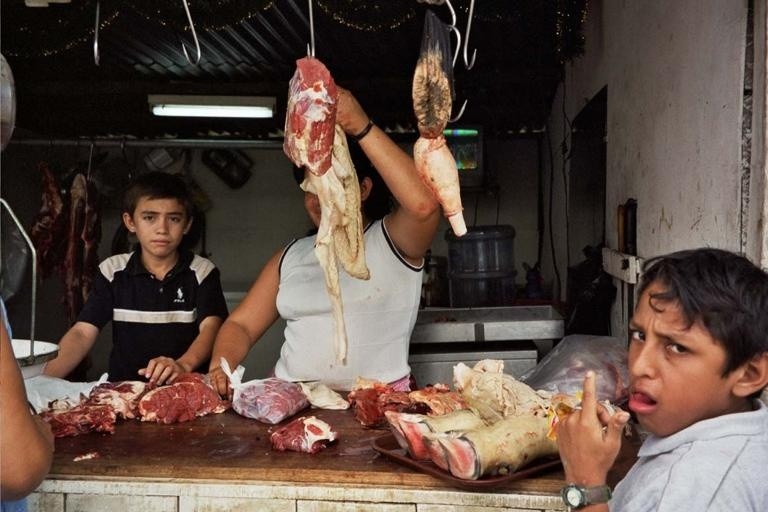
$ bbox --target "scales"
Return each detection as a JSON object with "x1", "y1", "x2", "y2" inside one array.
[{"x1": 0, "y1": 58, "x2": 59, "y2": 366}]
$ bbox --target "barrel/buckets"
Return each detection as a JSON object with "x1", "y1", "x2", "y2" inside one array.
[{"x1": 445, "y1": 224, "x2": 517, "y2": 308}]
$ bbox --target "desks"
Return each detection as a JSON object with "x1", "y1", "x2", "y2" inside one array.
[{"x1": 27, "y1": 390, "x2": 642, "y2": 512}]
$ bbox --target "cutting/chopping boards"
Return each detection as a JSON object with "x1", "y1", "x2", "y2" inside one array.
[{"x1": 50, "y1": 389, "x2": 647, "y2": 499}]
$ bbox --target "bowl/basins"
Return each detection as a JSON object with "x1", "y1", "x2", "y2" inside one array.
[{"x1": 12, "y1": 339, "x2": 60, "y2": 378}]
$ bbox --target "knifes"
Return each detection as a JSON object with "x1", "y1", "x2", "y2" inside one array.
[{"x1": 220, "y1": 378, "x2": 321, "y2": 399}]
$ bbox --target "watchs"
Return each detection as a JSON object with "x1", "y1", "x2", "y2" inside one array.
[{"x1": 556, "y1": 482, "x2": 613, "y2": 511}]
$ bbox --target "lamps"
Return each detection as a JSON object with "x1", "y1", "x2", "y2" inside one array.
[{"x1": 147, "y1": 94, "x2": 277, "y2": 120}]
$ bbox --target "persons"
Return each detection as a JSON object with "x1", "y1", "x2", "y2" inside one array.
[
  {"x1": 0, "y1": 293, "x2": 54, "y2": 512},
  {"x1": 551, "y1": 246, "x2": 766, "y2": 510},
  {"x1": 199, "y1": 82, "x2": 441, "y2": 399},
  {"x1": 40, "y1": 169, "x2": 231, "y2": 387}
]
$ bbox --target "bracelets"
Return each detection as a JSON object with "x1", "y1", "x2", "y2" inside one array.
[{"x1": 354, "y1": 118, "x2": 374, "y2": 142}]
